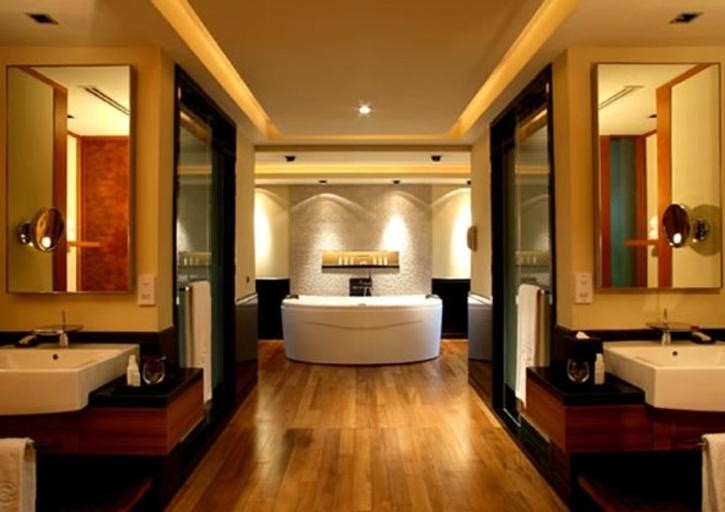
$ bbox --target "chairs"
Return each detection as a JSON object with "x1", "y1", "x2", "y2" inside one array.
[
  {"x1": 579, "y1": 471, "x2": 701, "y2": 511},
  {"x1": 35, "y1": 456, "x2": 152, "y2": 511}
]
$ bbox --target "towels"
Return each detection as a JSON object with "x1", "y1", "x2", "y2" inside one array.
[
  {"x1": 185, "y1": 280, "x2": 213, "y2": 404},
  {"x1": 0, "y1": 436, "x2": 36, "y2": 512},
  {"x1": 700, "y1": 432, "x2": 725, "y2": 511},
  {"x1": 514, "y1": 283, "x2": 546, "y2": 409}
]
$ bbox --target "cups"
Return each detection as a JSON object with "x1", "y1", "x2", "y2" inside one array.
[
  {"x1": 142, "y1": 362, "x2": 165, "y2": 385},
  {"x1": 567, "y1": 359, "x2": 590, "y2": 383}
]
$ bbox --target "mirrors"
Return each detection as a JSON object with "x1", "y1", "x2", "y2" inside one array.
[
  {"x1": 662, "y1": 203, "x2": 710, "y2": 248},
  {"x1": 17, "y1": 207, "x2": 65, "y2": 253},
  {"x1": 5, "y1": 63, "x2": 135, "y2": 294},
  {"x1": 591, "y1": 62, "x2": 724, "y2": 293}
]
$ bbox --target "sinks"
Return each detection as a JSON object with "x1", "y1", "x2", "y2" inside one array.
[
  {"x1": 0, "y1": 343, "x2": 141, "y2": 417},
  {"x1": 602, "y1": 338, "x2": 725, "y2": 413}
]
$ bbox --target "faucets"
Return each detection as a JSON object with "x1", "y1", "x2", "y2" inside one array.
[
  {"x1": 29, "y1": 327, "x2": 69, "y2": 348},
  {"x1": 645, "y1": 307, "x2": 672, "y2": 344}
]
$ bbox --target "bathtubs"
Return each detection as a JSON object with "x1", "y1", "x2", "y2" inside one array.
[{"x1": 280, "y1": 293, "x2": 444, "y2": 366}]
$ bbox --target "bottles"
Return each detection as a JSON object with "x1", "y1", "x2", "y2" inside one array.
[
  {"x1": 126, "y1": 353, "x2": 141, "y2": 387},
  {"x1": 594, "y1": 353, "x2": 605, "y2": 385}
]
